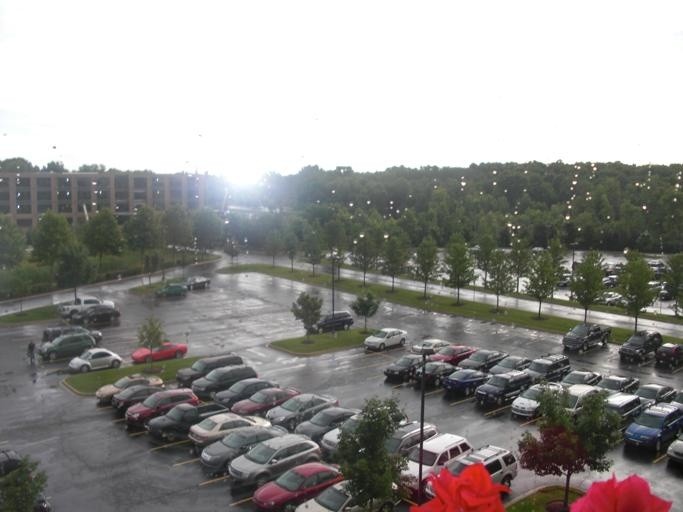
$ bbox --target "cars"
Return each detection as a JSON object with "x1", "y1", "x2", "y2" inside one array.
[
  {"x1": 551, "y1": 258, "x2": 675, "y2": 304},
  {"x1": 666, "y1": 433, "x2": 683, "y2": 462},
  {"x1": 384, "y1": 338, "x2": 677, "y2": 434},
  {"x1": 303, "y1": 310, "x2": 353, "y2": 336},
  {"x1": 95, "y1": 354, "x2": 363, "y2": 472},
  {"x1": 656, "y1": 343, "x2": 683, "y2": 367},
  {"x1": 363, "y1": 327, "x2": 407, "y2": 352},
  {"x1": 185, "y1": 276, "x2": 210, "y2": 290},
  {"x1": 37, "y1": 294, "x2": 122, "y2": 371},
  {"x1": 155, "y1": 283, "x2": 187, "y2": 298},
  {"x1": 251, "y1": 462, "x2": 346, "y2": 511},
  {"x1": 294, "y1": 479, "x2": 403, "y2": 512}
]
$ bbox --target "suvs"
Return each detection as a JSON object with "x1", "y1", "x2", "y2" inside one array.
[
  {"x1": 321, "y1": 407, "x2": 410, "y2": 456},
  {"x1": 562, "y1": 325, "x2": 612, "y2": 352},
  {"x1": 623, "y1": 401, "x2": 683, "y2": 453},
  {"x1": 425, "y1": 445, "x2": 519, "y2": 500},
  {"x1": 617, "y1": 330, "x2": 664, "y2": 365},
  {"x1": 358, "y1": 421, "x2": 439, "y2": 468},
  {"x1": 391, "y1": 432, "x2": 475, "y2": 505}
]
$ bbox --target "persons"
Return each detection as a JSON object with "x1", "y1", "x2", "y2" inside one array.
[{"x1": 27, "y1": 340, "x2": 36, "y2": 363}]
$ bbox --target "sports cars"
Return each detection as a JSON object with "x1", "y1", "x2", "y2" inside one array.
[{"x1": 132, "y1": 341, "x2": 188, "y2": 361}]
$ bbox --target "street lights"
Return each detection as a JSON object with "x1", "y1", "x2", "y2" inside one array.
[{"x1": 417, "y1": 349, "x2": 436, "y2": 506}]
